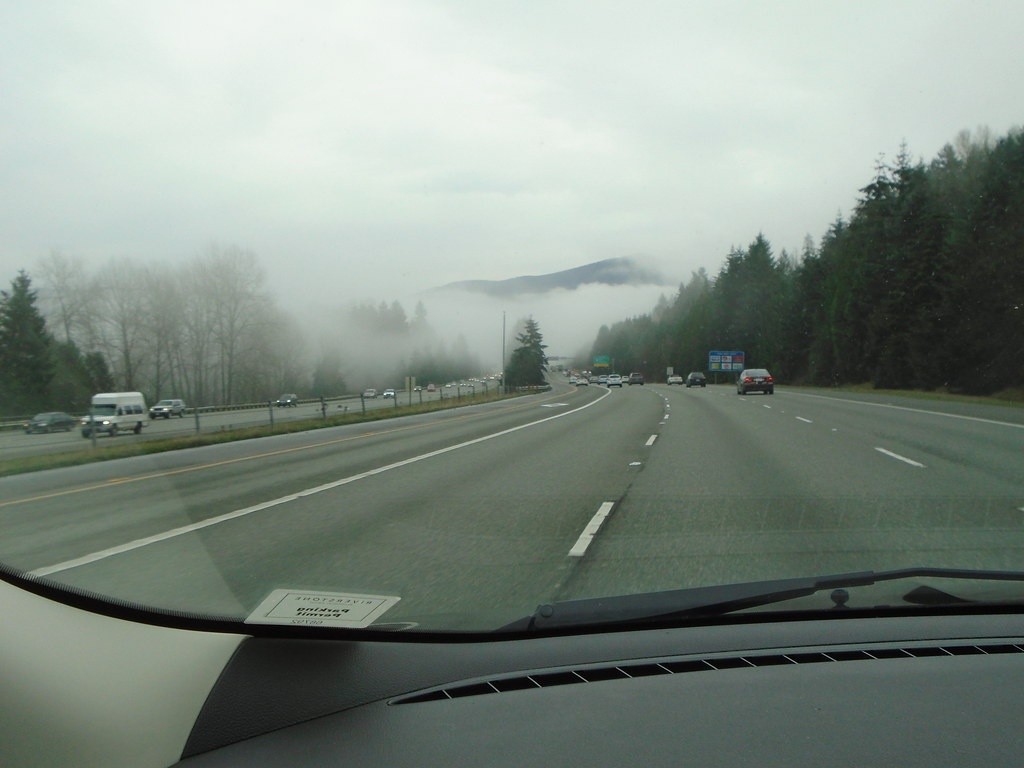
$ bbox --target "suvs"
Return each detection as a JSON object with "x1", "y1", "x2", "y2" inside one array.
[{"x1": 149, "y1": 398, "x2": 188, "y2": 418}]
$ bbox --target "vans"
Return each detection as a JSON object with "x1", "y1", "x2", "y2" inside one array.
[{"x1": 80, "y1": 391, "x2": 150, "y2": 438}]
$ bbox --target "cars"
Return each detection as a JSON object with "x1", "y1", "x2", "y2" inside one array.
[
  {"x1": 363, "y1": 388, "x2": 378, "y2": 399},
  {"x1": 621, "y1": 375, "x2": 629, "y2": 383},
  {"x1": 606, "y1": 374, "x2": 622, "y2": 388},
  {"x1": 627, "y1": 372, "x2": 644, "y2": 385},
  {"x1": 736, "y1": 367, "x2": 774, "y2": 396},
  {"x1": 667, "y1": 374, "x2": 683, "y2": 385},
  {"x1": 427, "y1": 383, "x2": 436, "y2": 392},
  {"x1": 276, "y1": 394, "x2": 298, "y2": 407},
  {"x1": 562, "y1": 367, "x2": 599, "y2": 386},
  {"x1": 23, "y1": 411, "x2": 78, "y2": 434},
  {"x1": 444, "y1": 370, "x2": 503, "y2": 388},
  {"x1": 686, "y1": 371, "x2": 706, "y2": 388},
  {"x1": 598, "y1": 374, "x2": 608, "y2": 385},
  {"x1": 413, "y1": 386, "x2": 422, "y2": 392},
  {"x1": 382, "y1": 388, "x2": 396, "y2": 399}
]
